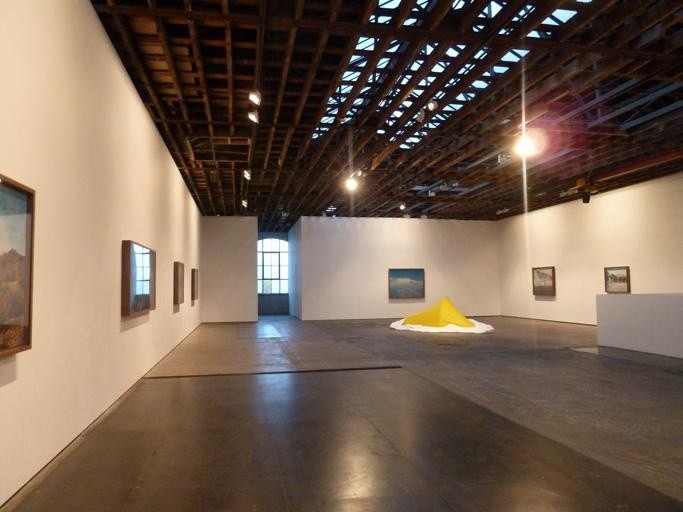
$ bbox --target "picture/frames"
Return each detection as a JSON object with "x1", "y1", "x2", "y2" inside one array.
[
  {"x1": 532, "y1": 265, "x2": 555, "y2": 296},
  {"x1": 603, "y1": 265, "x2": 630, "y2": 294},
  {"x1": 387, "y1": 267, "x2": 425, "y2": 299},
  {"x1": 0, "y1": 173, "x2": 35, "y2": 357},
  {"x1": 120, "y1": 239, "x2": 199, "y2": 360}
]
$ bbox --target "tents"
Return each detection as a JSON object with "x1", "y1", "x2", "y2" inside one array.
[{"x1": 390, "y1": 296, "x2": 494, "y2": 333}]
